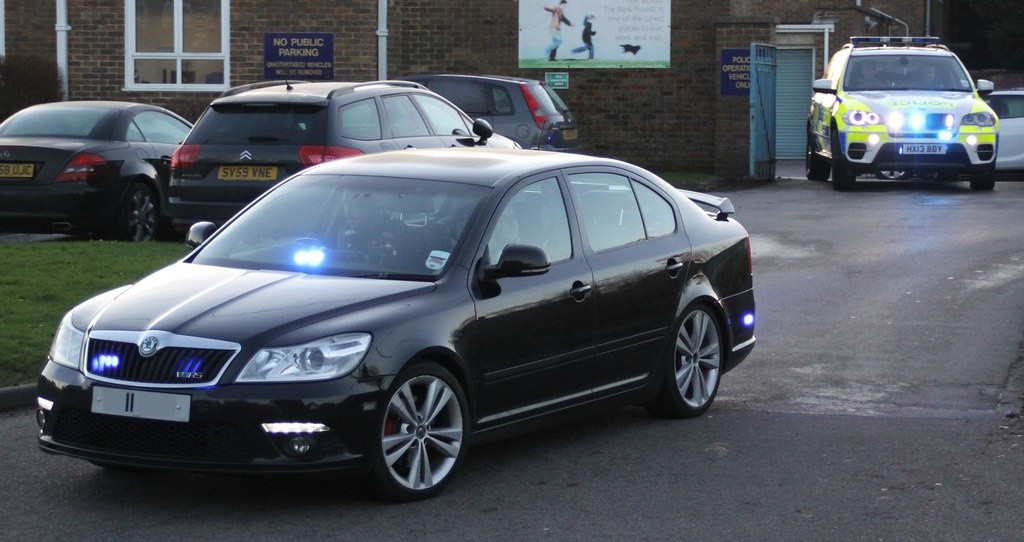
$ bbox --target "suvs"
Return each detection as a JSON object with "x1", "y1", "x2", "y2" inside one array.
[
  {"x1": 166, "y1": 78, "x2": 523, "y2": 238},
  {"x1": 805, "y1": 34, "x2": 1000, "y2": 192},
  {"x1": 391, "y1": 74, "x2": 583, "y2": 154}
]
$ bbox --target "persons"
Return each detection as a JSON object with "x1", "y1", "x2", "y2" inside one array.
[
  {"x1": 849, "y1": 61, "x2": 892, "y2": 88},
  {"x1": 914, "y1": 63, "x2": 946, "y2": 89}
]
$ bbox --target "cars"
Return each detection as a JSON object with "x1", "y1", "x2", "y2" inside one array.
[
  {"x1": 36, "y1": 145, "x2": 756, "y2": 501},
  {"x1": 987, "y1": 90, "x2": 1024, "y2": 180},
  {"x1": 0, "y1": 100, "x2": 195, "y2": 242}
]
